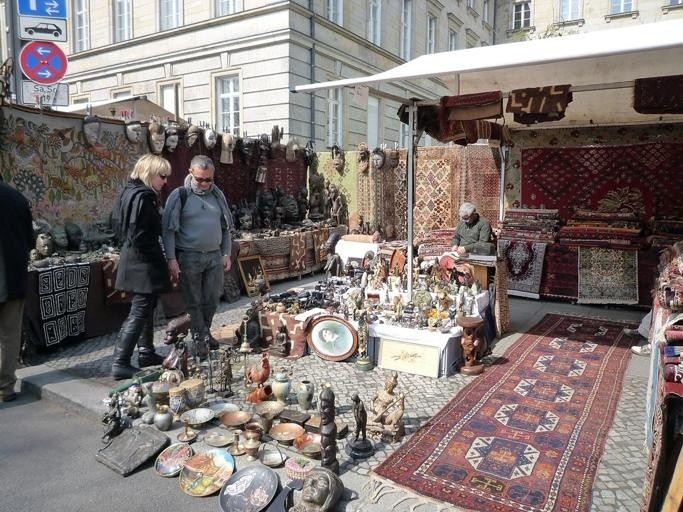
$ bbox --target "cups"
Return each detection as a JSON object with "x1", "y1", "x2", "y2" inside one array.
[
  {"x1": 260, "y1": 413, "x2": 273, "y2": 434},
  {"x1": 243, "y1": 437, "x2": 261, "y2": 462},
  {"x1": 242, "y1": 422, "x2": 263, "y2": 441}
]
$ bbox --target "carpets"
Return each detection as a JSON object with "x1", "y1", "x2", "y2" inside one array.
[
  {"x1": 487, "y1": 120, "x2": 502, "y2": 142},
  {"x1": 440, "y1": 89, "x2": 502, "y2": 124},
  {"x1": 540, "y1": 243, "x2": 579, "y2": 299},
  {"x1": 642, "y1": 241, "x2": 683, "y2": 512},
  {"x1": 396, "y1": 96, "x2": 439, "y2": 129},
  {"x1": 459, "y1": 120, "x2": 479, "y2": 147},
  {"x1": 553, "y1": 203, "x2": 646, "y2": 245},
  {"x1": 312, "y1": 149, "x2": 370, "y2": 235},
  {"x1": 630, "y1": 74, "x2": 683, "y2": 115},
  {"x1": 431, "y1": 104, "x2": 467, "y2": 145},
  {"x1": 461, "y1": 143, "x2": 500, "y2": 230},
  {"x1": 497, "y1": 205, "x2": 559, "y2": 245},
  {"x1": 381, "y1": 144, "x2": 461, "y2": 239},
  {"x1": 312, "y1": 226, "x2": 334, "y2": 263},
  {"x1": 576, "y1": 244, "x2": 640, "y2": 305},
  {"x1": 642, "y1": 212, "x2": 682, "y2": 245},
  {"x1": 101, "y1": 254, "x2": 136, "y2": 307},
  {"x1": 368, "y1": 310, "x2": 644, "y2": 512},
  {"x1": 30, "y1": 257, "x2": 93, "y2": 349},
  {"x1": 474, "y1": 117, "x2": 491, "y2": 139},
  {"x1": 496, "y1": 238, "x2": 547, "y2": 299},
  {"x1": 506, "y1": 84, "x2": 573, "y2": 126},
  {"x1": 163, "y1": 251, "x2": 184, "y2": 294},
  {"x1": 287, "y1": 232, "x2": 307, "y2": 273}
]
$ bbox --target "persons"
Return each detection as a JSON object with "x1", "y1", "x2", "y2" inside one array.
[
  {"x1": 36, "y1": 232, "x2": 54, "y2": 257},
  {"x1": 318, "y1": 328, "x2": 350, "y2": 350},
  {"x1": 328, "y1": 188, "x2": 343, "y2": 225},
  {"x1": 349, "y1": 394, "x2": 368, "y2": 444},
  {"x1": 112, "y1": 155, "x2": 173, "y2": 373},
  {"x1": 161, "y1": 154, "x2": 233, "y2": 349},
  {"x1": 452, "y1": 203, "x2": 491, "y2": 257},
  {"x1": 369, "y1": 373, "x2": 409, "y2": 430},
  {"x1": 235, "y1": 201, "x2": 255, "y2": 229},
  {"x1": 290, "y1": 471, "x2": 329, "y2": 512},
  {"x1": 0, "y1": 177, "x2": 33, "y2": 403}
]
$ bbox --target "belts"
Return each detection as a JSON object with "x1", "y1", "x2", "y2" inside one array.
[{"x1": 175, "y1": 248, "x2": 222, "y2": 255}]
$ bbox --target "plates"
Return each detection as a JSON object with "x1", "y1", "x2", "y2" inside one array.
[
  {"x1": 268, "y1": 422, "x2": 306, "y2": 446},
  {"x1": 220, "y1": 410, "x2": 251, "y2": 430},
  {"x1": 179, "y1": 447, "x2": 235, "y2": 497},
  {"x1": 255, "y1": 401, "x2": 284, "y2": 416},
  {"x1": 227, "y1": 444, "x2": 247, "y2": 456},
  {"x1": 154, "y1": 442, "x2": 193, "y2": 478},
  {"x1": 203, "y1": 429, "x2": 234, "y2": 446},
  {"x1": 209, "y1": 403, "x2": 240, "y2": 419},
  {"x1": 217, "y1": 464, "x2": 279, "y2": 512},
  {"x1": 180, "y1": 408, "x2": 214, "y2": 430},
  {"x1": 177, "y1": 431, "x2": 198, "y2": 445}
]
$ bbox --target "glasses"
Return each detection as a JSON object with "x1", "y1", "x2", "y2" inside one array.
[
  {"x1": 191, "y1": 172, "x2": 214, "y2": 183},
  {"x1": 158, "y1": 174, "x2": 167, "y2": 180}
]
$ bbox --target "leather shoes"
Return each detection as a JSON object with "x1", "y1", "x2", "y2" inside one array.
[{"x1": 191, "y1": 333, "x2": 219, "y2": 350}]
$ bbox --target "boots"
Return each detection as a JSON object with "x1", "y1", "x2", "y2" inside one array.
[
  {"x1": 110, "y1": 317, "x2": 143, "y2": 381},
  {"x1": 138, "y1": 311, "x2": 166, "y2": 367}
]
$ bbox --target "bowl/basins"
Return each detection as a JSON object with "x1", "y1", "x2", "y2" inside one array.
[{"x1": 292, "y1": 432, "x2": 322, "y2": 458}]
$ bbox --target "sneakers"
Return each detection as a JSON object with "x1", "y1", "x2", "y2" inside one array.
[{"x1": 0, "y1": 391, "x2": 16, "y2": 402}]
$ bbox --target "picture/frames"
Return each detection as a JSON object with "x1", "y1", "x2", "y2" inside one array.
[
  {"x1": 304, "y1": 315, "x2": 358, "y2": 361},
  {"x1": 235, "y1": 254, "x2": 271, "y2": 297}
]
